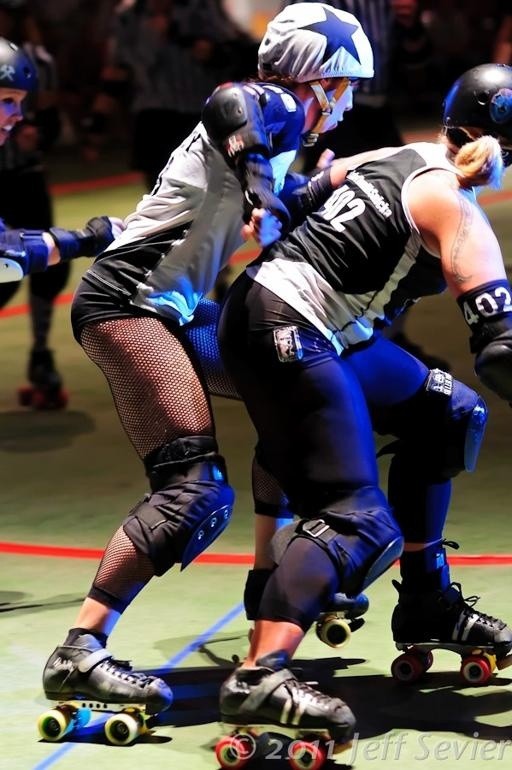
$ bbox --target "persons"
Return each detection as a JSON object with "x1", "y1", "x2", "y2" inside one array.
[
  {"x1": 218, "y1": 62, "x2": 512, "y2": 731},
  {"x1": 42, "y1": 2, "x2": 374, "y2": 714},
  {"x1": 0, "y1": 1, "x2": 511, "y2": 412}
]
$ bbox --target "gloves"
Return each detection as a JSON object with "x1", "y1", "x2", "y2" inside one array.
[
  {"x1": 47, "y1": 213, "x2": 116, "y2": 266},
  {"x1": 237, "y1": 160, "x2": 293, "y2": 240}
]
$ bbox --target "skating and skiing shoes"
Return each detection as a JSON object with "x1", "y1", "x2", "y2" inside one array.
[
  {"x1": 212, "y1": 651, "x2": 359, "y2": 770},
  {"x1": 388, "y1": 579, "x2": 512, "y2": 685},
  {"x1": 17, "y1": 349, "x2": 71, "y2": 414},
  {"x1": 242, "y1": 568, "x2": 369, "y2": 650},
  {"x1": 38, "y1": 634, "x2": 174, "y2": 747}
]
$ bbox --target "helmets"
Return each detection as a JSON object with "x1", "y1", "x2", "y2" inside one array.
[
  {"x1": 443, "y1": 63, "x2": 512, "y2": 151},
  {"x1": 256, "y1": 2, "x2": 374, "y2": 86},
  {"x1": 1, "y1": 35, "x2": 39, "y2": 91}
]
